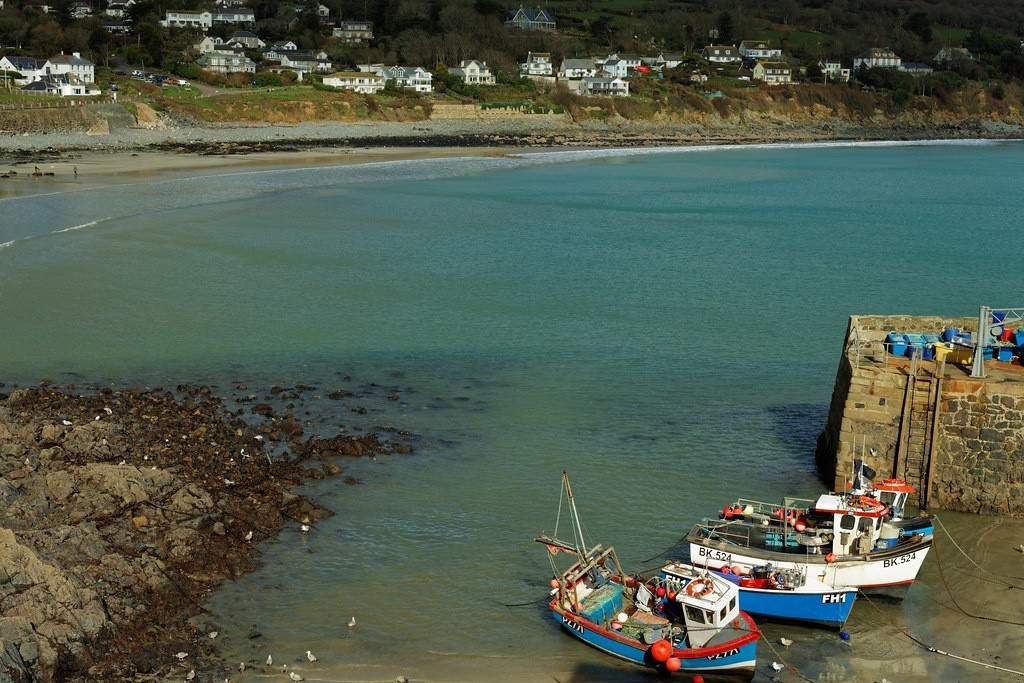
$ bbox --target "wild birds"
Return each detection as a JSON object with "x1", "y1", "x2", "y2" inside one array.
[
  {"x1": 780, "y1": 637, "x2": 795, "y2": 648},
  {"x1": 772, "y1": 662, "x2": 784, "y2": 673},
  {"x1": 173, "y1": 632, "x2": 318, "y2": 682},
  {"x1": 347, "y1": 616, "x2": 356, "y2": 627}
]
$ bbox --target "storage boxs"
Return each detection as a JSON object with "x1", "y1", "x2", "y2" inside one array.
[
  {"x1": 978, "y1": 327, "x2": 1024, "y2": 364},
  {"x1": 905, "y1": 334, "x2": 927, "y2": 358},
  {"x1": 887, "y1": 333, "x2": 910, "y2": 356},
  {"x1": 922, "y1": 334, "x2": 944, "y2": 359},
  {"x1": 572, "y1": 580, "x2": 625, "y2": 626}
]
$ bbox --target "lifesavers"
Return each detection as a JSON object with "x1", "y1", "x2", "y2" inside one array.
[
  {"x1": 847, "y1": 498, "x2": 871, "y2": 508},
  {"x1": 858, "y1": 495, "x2": 880, "y2": 508},
  {"x1": 882, "y1": 477, "x2": 905, "y2": 484},
  {"x1": 686, "y1": 579, "x2": 713, "y2": 598}
]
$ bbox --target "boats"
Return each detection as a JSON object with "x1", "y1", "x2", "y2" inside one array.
[
  {"x1": 532, "y1": 471, "x2": 759, "y2": 682},
  {"x1": 686, "y1": 432, "x2": 932, "y2": 605},
  {"x1": 658, "y1": 561, "x2": 858, "y2": 633}
]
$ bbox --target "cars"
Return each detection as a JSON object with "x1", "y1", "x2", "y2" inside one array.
[{"x1": 115, "y1": 69, "x2": 192, "y2": 89}]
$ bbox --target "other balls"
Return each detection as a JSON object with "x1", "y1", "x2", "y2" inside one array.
[
  {"x1": 657, "y1": 586, "x2": 666, "y2": 597},
  {"x1": 691, "y1": 674, "x2": 705, "y2": 683},
  {"x1": 666, "y1": 655, "x2": 681, "y2": 673},
  {"x1": 669, "y1": 588, "x2": 679, "y2": 600},
  {"x1": 840, "y1": 631, "x2": 850, "y2": 641},
  {"x1": 771, "y1": 505, "x2": 807, "y2": 532},
  {"x1": 651, "y1": 639, "x2": 673, "y2": 662},
  {"x1": 723, "y1": 501, "x2": 754, "y2": 518},
  {"x1": 618, "y1": 612, "x2": 629, "y2": 623},
  {"x1": 574, "y1": 602, "x2": 583, "y2": 611},
  {"x1": 550, "y1": 579, "x2": 560, "y2": 589},
  {"x1": 721, "y1": 564, "x2": 731, "y2": 574},
  {"x1": 611, "y1": 619, "x2": 623, "y2": 631},
  {"x1": 731, "y1": 566, "x2": 741, "y2": 576},
  {"x1": 825, "y1": 552, "x2": 838, "y2": 563}
]
